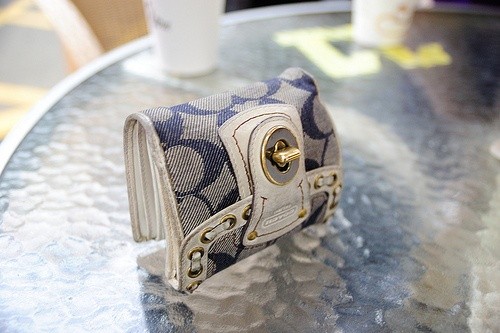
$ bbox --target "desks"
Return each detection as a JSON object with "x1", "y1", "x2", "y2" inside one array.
[{"x1": 0, "y1": 0, "x2": 500, "y2": 333}]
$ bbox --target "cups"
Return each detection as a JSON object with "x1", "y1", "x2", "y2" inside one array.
[
  {"x1": 142, "y1": 0, "x2": 226, "y2": 77},
  {"x1": 350, "y1": 0, "x2": 416, "y2": 48}
]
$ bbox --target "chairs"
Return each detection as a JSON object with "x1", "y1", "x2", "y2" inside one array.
[{"x1": 0, "y1": 0, "x2": 105, "y2": 143}]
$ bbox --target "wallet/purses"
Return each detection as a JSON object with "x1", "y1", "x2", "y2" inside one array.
[{"x1": 117, "y1": 64, "x2": 344, "y2": 294}]
